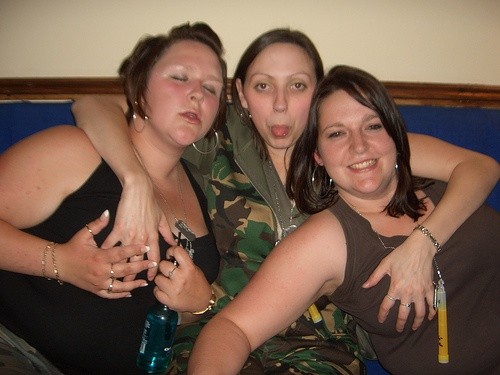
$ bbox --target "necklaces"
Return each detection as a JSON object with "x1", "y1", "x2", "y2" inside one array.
[
  {"x1": 268, "y1": 159, "x2": 297, "y2": 248},
  {"x1": 341, "y1": 192, "x2": 445, "y2": 289}
]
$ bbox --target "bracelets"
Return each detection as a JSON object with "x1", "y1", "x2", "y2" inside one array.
[
  {"x1": 413, "y1": 224, "x2": 442, "y2": 251},
  {"x1": 41, "y1": 242, "x2": 64, "y2": 285},
  {"x1": 192, "y1": 286, "x2": 216, "y2": 315}
]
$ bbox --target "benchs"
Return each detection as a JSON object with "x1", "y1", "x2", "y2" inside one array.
[{"x1": 0, "y1": 77, "x2": 500, "y2": 375}]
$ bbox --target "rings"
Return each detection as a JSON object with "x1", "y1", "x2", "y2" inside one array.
[
  {"x1": 110, "y1": 263, "x2": 115, "y2": 278},
  {"x1": 386, "y1": 294, "x2": 396, "y2": 302},
  {"x1": 168, "y1": 266, "x2": 177, "y2": 278},
  {"x1": 108, "y1": 279, "x2": 114, "y2": 291},
  {"x1": 86, "y1": 224, "x2": 95, "y2": 236},
  {"x1": 399, "y1": 303, "x2": 412, "y2": 308}
]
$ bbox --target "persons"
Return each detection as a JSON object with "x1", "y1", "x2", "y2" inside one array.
[
  {"x1": 0, "y1": 22, "x2": 229, "y2": 375},
  {"x1": 188, "y1": 65, "x2": 500, "y2": 375},
  {"x1": 71, "y1": 28, "x2": 500, "y2": 375}
]
